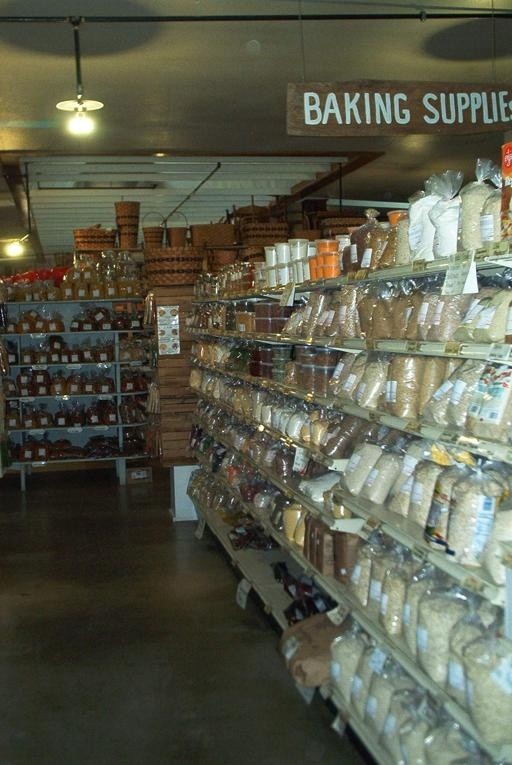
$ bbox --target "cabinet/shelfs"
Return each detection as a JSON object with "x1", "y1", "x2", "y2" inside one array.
[{"x1": 0, "y1": 245, "x2": 512, "y2": 765}]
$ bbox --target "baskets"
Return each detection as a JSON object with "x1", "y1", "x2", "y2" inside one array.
[
  {"x1": 207, "y1": 244, "x2": 239, "y2": 274},
  {"x1": 114, "y1": 198, "x2": 140, "y2": 250},
  {"x1": 143, "y1": 246, "x2": 205, "y2": 290},
  {"x1": 72, "y1": 223, "x2": 117, "y2": 269},
  {"x1": 190, "y1": 223, "x2": 238, "y2": 246},
  {"x1": 167, "y1": 227, "x2": 189, "y2": 246},
  {"x1": 239, "y1": 242, "x2": 275, "y2": 262},
  {"x1": 143, "y1": 227, "x2": 165, "y2": 245},
  {"x1": 237, "y1": 205, "x2": 372, "y2": 243}
]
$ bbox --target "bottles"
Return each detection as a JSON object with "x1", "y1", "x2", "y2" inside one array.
[{"x1": 193, "y1": 262, "x2": 258, "y2": 299}]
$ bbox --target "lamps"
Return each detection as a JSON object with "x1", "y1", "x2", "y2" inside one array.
[{"x1": 55, "y1": 15, "x2": 105, "y2": 137}]
